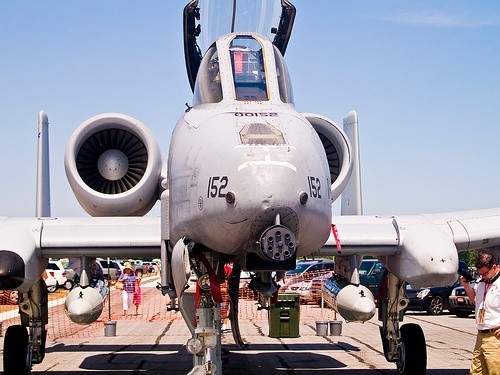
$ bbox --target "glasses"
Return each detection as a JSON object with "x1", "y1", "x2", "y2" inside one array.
[{"x1": 479, "y1": 269, "x2": 490, "y2": 276}]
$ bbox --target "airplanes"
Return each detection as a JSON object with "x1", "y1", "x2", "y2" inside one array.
[{"x1": 0, "y1": 1, "x2": 500, "y2": 375}]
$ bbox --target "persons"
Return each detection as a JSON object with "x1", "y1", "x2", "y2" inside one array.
[
  {"x1": 220, "y1": 265, "x2": 232, "y2": 329},
  {"x1": 118, "y1": 262, "x2": 136, "y2": 316},
  {"x1": 72, "y1": 267, "x2": 79, "y2": 284},
  {"x1": 460, "y1": 250, "x2": 500, "y2": 375}
]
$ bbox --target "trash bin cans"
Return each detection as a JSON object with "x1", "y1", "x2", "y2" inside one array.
[{"x1": 268, "y1": 292, "x2": 301, "y2": 338}]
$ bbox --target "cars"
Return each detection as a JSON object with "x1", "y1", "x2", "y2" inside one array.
[
  {"x1": 238, "y1": 257, "x2": 481, "y2": 319},
  {"x1": 0, "y1": 257, "x2": 161, "y2": 305}
]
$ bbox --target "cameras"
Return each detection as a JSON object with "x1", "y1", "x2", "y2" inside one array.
[{"x1": 458, "y1": 264, "x2": 472, "y2": 283}]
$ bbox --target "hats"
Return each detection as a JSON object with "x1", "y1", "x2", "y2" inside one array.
[{"x1": 123, "y1": 262, "x2": 134, "y2": 270}]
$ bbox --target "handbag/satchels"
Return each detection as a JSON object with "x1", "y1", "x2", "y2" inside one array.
[{"x1": 115, "y1": 280, "x2": 123, "y2": 289}]
